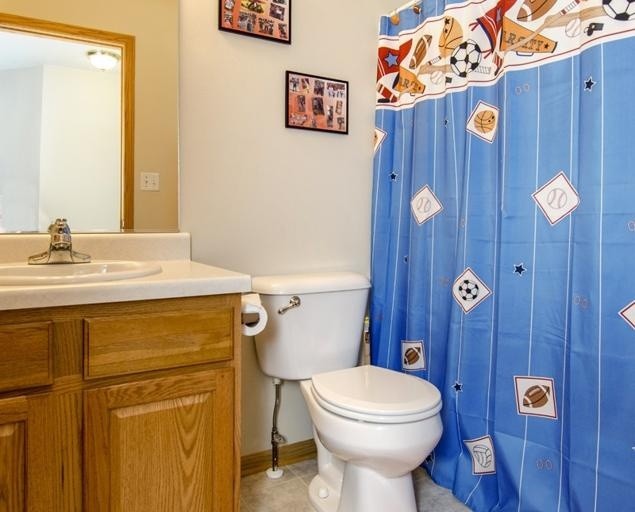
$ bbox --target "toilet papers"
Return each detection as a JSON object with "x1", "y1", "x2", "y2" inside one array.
[{"x1": 241, "y1": 293, "x2": 268, "y2": 337}]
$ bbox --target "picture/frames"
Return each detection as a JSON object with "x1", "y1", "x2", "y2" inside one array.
[
  {"x1": 218, "y1": 0, "x2": 294, "y2": 46},
  {"x1": 283, "y1": 70, "x2": 350, "y2": 136}
]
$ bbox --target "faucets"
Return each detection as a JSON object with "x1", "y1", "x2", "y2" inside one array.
[{"x1": 28, "y1": 219, "x2": 92, "y2": 265}]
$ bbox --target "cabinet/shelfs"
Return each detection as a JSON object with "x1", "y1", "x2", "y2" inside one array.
[{"x1": 1, "y1": 287, "x2": 243, "y2": 511}]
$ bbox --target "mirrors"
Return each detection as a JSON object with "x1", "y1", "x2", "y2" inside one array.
[{"x1": 0, "y1": 14, "x2": 135, "y2": 234}]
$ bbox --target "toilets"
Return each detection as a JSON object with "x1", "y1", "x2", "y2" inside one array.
[{"x1": 253, "y1": 273, "x2": 444, "y2": 512}]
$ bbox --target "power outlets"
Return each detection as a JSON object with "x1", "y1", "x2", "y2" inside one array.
[{"x1": 139, "y1": 172, "x2": 159, "y2": 192}]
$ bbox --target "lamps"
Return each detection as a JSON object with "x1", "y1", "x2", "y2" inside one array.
[{"x1": 86, "y1": 49, "x2": 121, "y2": 73}]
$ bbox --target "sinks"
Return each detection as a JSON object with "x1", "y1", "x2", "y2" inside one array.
[{"x1": 0, "y1": 260, "x2": 162, "y2": 284}]
